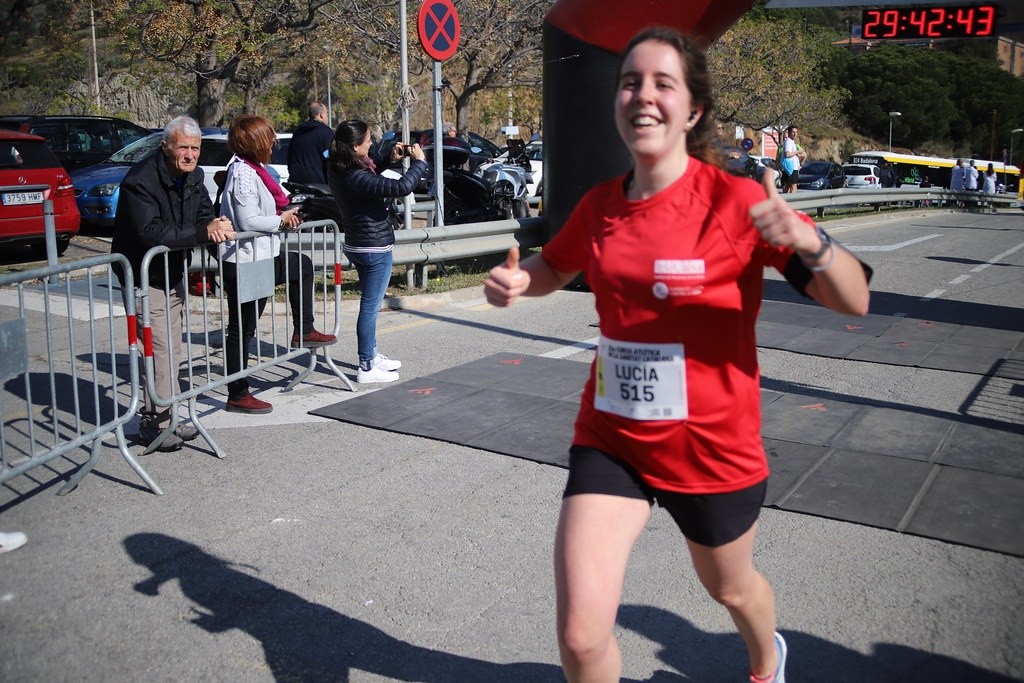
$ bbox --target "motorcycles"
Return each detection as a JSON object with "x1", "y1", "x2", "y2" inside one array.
[
  {"x1": 285, "y1": 132, "x2": 407, "y2": 233},
  {"x1": 420, "y1": 130, "x2": 543, "y2": 228}
]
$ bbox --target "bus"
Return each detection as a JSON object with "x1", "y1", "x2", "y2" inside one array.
[{"x1": 847, "y1": 151, "x2": 1024, "y2": 204}]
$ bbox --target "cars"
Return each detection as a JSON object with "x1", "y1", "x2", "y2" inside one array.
[
  {"x1": 69, "y1": 128, "x2": 230, "y2": 226},
  {"x1": 196, "y1": 132, "x2": 300, "y2": 207},
  {"x1": 797, "y1": 161, "x2": 848, "y2": 191},
  {"x1": 840, "y1": 163, "x2": 882, "y2": 189},
  {"x1": 377, "y1": 125, "x2": 544, "y2": 201},
  {"x1": 0, "y1": 129, "x2": 80, "y2": 259},
  {"x1": 719, "y1": 143, "x2": 782, "y2": 187}
]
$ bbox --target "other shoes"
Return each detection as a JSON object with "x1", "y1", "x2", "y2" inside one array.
[
  {"x1": 226, "y1": 391, "x2": 274, "y2": 414},
  {"x1": 291, "y1": 329, "x2": 334, "y2": 348}
]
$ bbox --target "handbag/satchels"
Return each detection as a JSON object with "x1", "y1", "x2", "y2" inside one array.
[{"x1": 779, "y1": 155, "x2": 794, "y2": 177}]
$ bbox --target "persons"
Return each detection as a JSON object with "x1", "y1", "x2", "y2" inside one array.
[
  {"x1": 949, "y1": 159, "x2": 997, "y2": 207},
  {"x1": 443, "y1": 122, "x2": 456, "y2": 137},
  {"x1": 111, "y1": 118, "x2": 236, "y2": 452},
  {"x1": 485, "y1": 30, "x2": 870, "y2": 683},
  {"x1": 287, "y1": 102, "x2": 333, "y2": 184},
  {"x1": 329, "y1": 119, "x2": 428, "y2": 383},
  {"x1": 879, "y1": 161, "x2": 902, "y2": 188},
  {"x1": 220, "y1": 117, "x2": 337, "y2": 414},
  {"x1": 776, "y1": 125, "x2": 807, "y2": 193},
  {"x1": 920, "y1": 176, "x2": 931, "y2": 189},
  {"x1": 713, "y1": 121, "x2": 741, "y2": 159}
]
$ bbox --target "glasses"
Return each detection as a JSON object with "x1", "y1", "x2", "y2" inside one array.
[{"x1": 452, "y1": 130, "x2": 457, "y2": 133}]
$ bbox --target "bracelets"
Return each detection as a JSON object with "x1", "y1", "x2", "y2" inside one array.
[
  {"x1": 804, "y1": 245, "x2": 835, "y2": 271},
  {"x1": 800, "y1": 164, "x2": 803, "y2": 166}
]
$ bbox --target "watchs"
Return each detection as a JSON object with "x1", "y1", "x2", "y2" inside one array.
[
  {"x1": 279, "y1": 218, "x2": 285, "y2": 230},
  {"x1": 797, "y1": 225, "x2": 830, "y2": 260}
]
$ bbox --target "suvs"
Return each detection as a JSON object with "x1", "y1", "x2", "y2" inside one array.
[{"x1": 0, "y1": 113, "x2": 156, "y2": 174}]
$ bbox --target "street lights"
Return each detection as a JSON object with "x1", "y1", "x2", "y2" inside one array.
[
  {"x1": 1010, "y1": 128, "x2": 1023, "y2": 165},
  {"x1": 889, "y1": 112, "x2": 901, "y2": 152}
]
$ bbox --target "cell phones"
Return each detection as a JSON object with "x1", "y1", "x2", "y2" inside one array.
[{"x1": 403, "y1": 145, "x2": 412, "y2": 157}]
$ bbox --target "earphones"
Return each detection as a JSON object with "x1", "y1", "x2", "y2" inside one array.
[{"x1": 688, "y1": 109, "x2": 698, "y2": 121}]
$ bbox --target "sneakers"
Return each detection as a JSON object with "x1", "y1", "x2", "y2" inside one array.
[
  {"x1": 173, "y1": 425, "x2": 199, "y2": 441},
  {"x1": 748, "y1": 630, "x2": 788, "y2": 683},
  {"x1": 356, "y1": 365, "x2": 400, "y2": 384},
  {"x1": 373, "y1": 353, "x2": 401, "y2": 370},
  {"x1": 136, "y1": 425, "x2": 184, "y2": 453}
]
